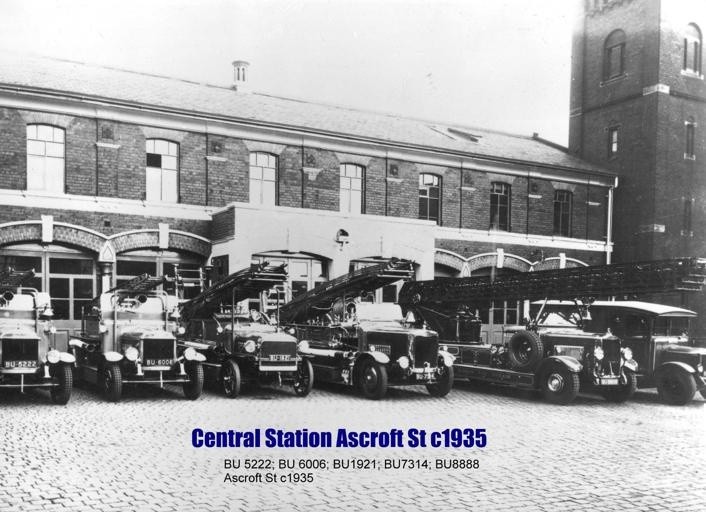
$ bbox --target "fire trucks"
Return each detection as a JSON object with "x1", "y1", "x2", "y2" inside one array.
[{"x1": 0, "y1": 249, "x2": 706, "y2": 410}]
